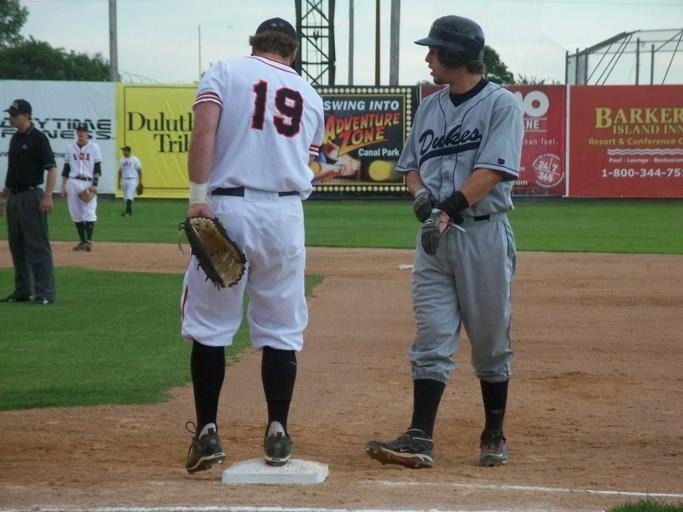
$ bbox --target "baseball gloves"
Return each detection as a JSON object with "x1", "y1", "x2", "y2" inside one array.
[
  {"x1": 136, "y1": 184, "x2": 143, "y2": 196},
  {"x1": 183, "y1": 216, "x2": 246, "y2": 288},
  {"x1": 79, "y1": 187, "x2": 96, "y2": 202}
]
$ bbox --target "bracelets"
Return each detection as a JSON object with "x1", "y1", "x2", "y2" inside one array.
[
  {"x1": 436, "y1": 190, "x2": 470, "y2": 218},
  {"x1": 91, "y1": 176, "x2": 99, "y2": 187},
  {"x1": 185, "y1": 179, "x2": 208, "y2": 206}
]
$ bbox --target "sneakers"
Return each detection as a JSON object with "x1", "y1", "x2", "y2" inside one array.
[
  {"x1": 120, "y1": 210, "x2": 131, "y2": 217},
  {"x1": 0, "y1": 293, "x2": 53, "y2": 307},
  {"x1": 261, "y1": 432, "x2": 294, "y2": 468},
  {"x1": 363, "y1": 425, "x2": 435, "y2": 471},
  {"x1": 183, "y1": 419, "x2": 226, "y2": 477},
  {"x1": 71, "y1": 238, "x2": 92, "y2": 252},
  {"x1": 477, "y1": 428, "x2": 508, "y2": 468}
]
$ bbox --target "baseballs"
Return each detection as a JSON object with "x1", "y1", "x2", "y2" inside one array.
[{"x1": 369, "y1": 161, "x2": 390, "y2": 181}]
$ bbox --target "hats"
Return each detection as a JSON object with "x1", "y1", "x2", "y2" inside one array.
[
  {"x1": 3, "y1": 98, "x2": 33, "y2": 116},
  {"x1": 119, "y1": 146, "x2": 131, "y2": 152},
  {"x1": 255, "y1": 16, "x2": 298, "y2": 41},
  {"x1": 75, "y1": 122, "x2": 89, "y2": 131}
]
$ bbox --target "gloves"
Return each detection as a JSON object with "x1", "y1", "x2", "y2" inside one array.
[
  {"x1": 419, "y1": 206, "x2": 450, "y2": 257},
  {"x1": 412, "y1": 186, "x2": 440, "y2": 225}
]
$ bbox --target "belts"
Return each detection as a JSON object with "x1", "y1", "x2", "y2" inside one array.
[
  {"x1": 10, "y1": 185, "x2": 38, "y2": 194},
  {"x1": 209, "y1": 185, "x2": 300, "y2": 200},
  {"x1": 70, "y1": 175, "x2": 92, "y2": 182},
  {"x1": 122, "y1": 176, "x2": 136, "y2": 179}
]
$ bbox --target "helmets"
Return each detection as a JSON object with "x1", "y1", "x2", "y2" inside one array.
[{"x1": 412, "y1": 14, "x2": 485, "y2": 64}]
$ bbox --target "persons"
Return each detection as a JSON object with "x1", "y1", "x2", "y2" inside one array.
[
  {"x1": 116, "y1": 145, "x2": 143, "y2": 217},
  {"x1": 360, "y1": 12, "x2": 524, "y2": 471},
  {"x1": 60, "y1": 122, "x2": 101, "y2": 252},
  {"x1": 175, "y1": 15, "x2": 326, "y2": 478},
  {"x1": 0, "y1": 98, "x2": 59, "y2": 307}
]
